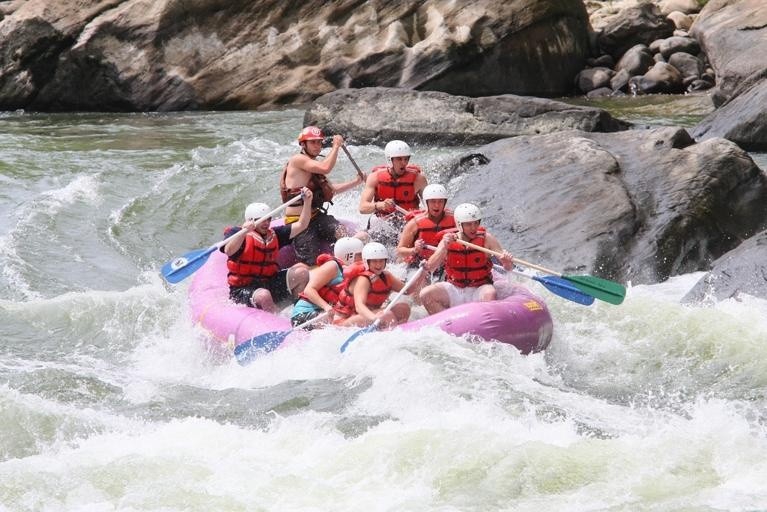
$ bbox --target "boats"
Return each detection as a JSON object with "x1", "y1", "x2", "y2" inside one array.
[{"x1": 179, "y1": 212, "x2": 558, "y2": 372}]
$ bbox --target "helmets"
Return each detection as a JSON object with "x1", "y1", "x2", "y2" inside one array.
[
  {"x1": 298, "y1": 125, "x2": 324, "y2": 141},
  {"x1": 422, "y1": 183, "x2": 449, "y2": 200},
  {"x1": 333, "y1": 236, "x2": 364, "y2": 254},
  {"x1": 384, "y1": 139, "x2": 412, "y2": 158},
  {"x1": 244, "y1": 202, "x2": 273, "y2": 220},
  {"x1": 361, "y1": 241, "x2": 389, "y2": 260},
  {"x1": 453, "y1": 202, "x2": 482, "y2": 223}
]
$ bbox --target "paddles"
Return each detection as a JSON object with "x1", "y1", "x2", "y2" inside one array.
[
  {"x1": 161, "y1": 193, "x2": 305, "y2": 284},
  {"x1": 340, "y1": 265, "x2": 425, "y2": 354},
  {"x1": 421, "y1": 237, "x2": 626, "y2": 306},
  {"x1": 234, "y1": 311, "x2": 327, "y2": 369}
]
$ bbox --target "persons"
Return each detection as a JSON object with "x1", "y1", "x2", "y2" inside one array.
[
  {"x1": 219, "y1": 185, "x2": 314, "y2": 315},
  {"x1": 290, "y1": 236, "x2": 364, "y2": 330},
  {"x1": 332, "y1": 243, "x2": 410, "y2": 330},
  {"x1": 280, "y1": 126, "x2": 368, "y2": 268},
  {"x1": 396, "y1": 184, "x2": 455, "y2": 305},
  {"x1": 419, "y1": 204, "x2": 514, "y2": 316},
  {"x1": 355, "y1": 139, "x2": 429, "y2": 246}
]
